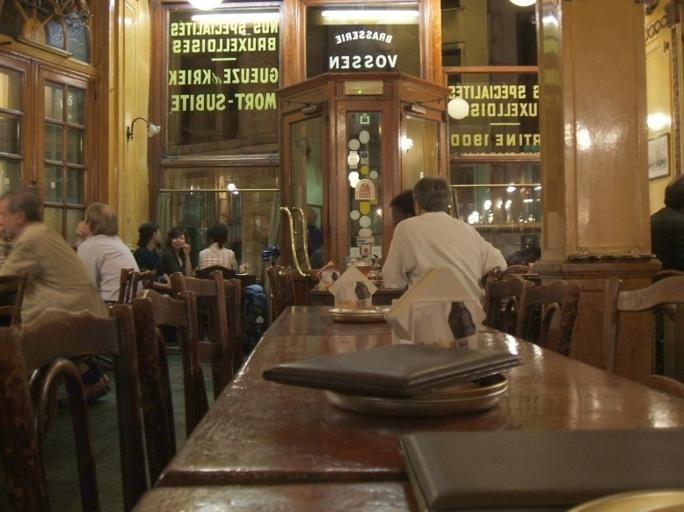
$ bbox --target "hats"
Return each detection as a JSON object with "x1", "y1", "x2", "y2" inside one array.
[{"x1": 136, "y1": 223, "x2": 159, "y2": 246}]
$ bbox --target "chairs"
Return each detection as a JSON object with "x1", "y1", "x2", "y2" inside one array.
[
  {"x1": 170, "y1": 270, "x2": 241, "y2": 442},
  {"x1": 119, "y1": 267, "x2": 156, "y2": 303},
  {"x1": 133, "y1": 288, "x2": 210, "y2": 483},
  {"x1": 266, "y1": 264, "x2": 296, "y2": 321},
  {"x1": 604, "y1": 274, "x2": 682, "y2": 397},
  {"x1": 524, "y1": 282, "x2": 614, "y2": 369},
  {"x1": 485, "y1": 274, "x2": 539, "y2": 340},
  {"x1": 195, "y1": 266, "x2": 236, "y2": 344},
  {"x1": 1, "y1": 304, "x2": 149, "y2": 512}
]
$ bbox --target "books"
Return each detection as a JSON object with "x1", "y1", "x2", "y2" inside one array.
[
  {"x1": 262, "y1": 342, "x2": 524, "y2": 399},
  {"x1": 395, "y1": 425, "x2": 684, "y2": 511}
]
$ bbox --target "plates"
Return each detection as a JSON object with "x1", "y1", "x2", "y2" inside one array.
[
  {"x1": 326, "y1": 305, "x2": 388, "y2": 316},
  {"x1": 321, "y1": 372, "x2": 509, "y2": 417},
  {"x1": 325, "y1": 315, "x2": 390, "y2": 323}
]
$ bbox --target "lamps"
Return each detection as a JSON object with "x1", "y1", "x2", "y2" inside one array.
[
  {"x1": 128, "y1": 116, "x2": 162, "y2": 140},
  {"x1": 447, "y1": 96, "x2": 470, "y2": 119}
]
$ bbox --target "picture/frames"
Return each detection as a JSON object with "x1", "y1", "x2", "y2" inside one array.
[{"x1": 649, "y1": 133, "x2": 670, "y2": 179}]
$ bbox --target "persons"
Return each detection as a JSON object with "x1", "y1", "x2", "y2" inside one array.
[
  {"x1": 198, "y1": 224, "x2": 240, "y2": 279},
  {"x1": 160, "y1": 225, "x2": 195, "y2": 280},
  {"x1": 388, "y1": 190, "x2": 416, "y2": 227},
  {"x1": 651, "y1": 173, "x2": 683, "y2": 268},
  {"x1": 134, "y1": 223, "x2": 181, "y2": 343},
  {"x1": 63, "y1": 202, "x2": 144, "y2": 402},
  {"x1": 302, "y1": 206, "x2": 324, "y2": 268},
  {"x1": 382, "y1": 177, "x2": 509, "y2": 323},
  {"x1": 1, "y1": 188, "x2": 111, "y2": 332}
]
